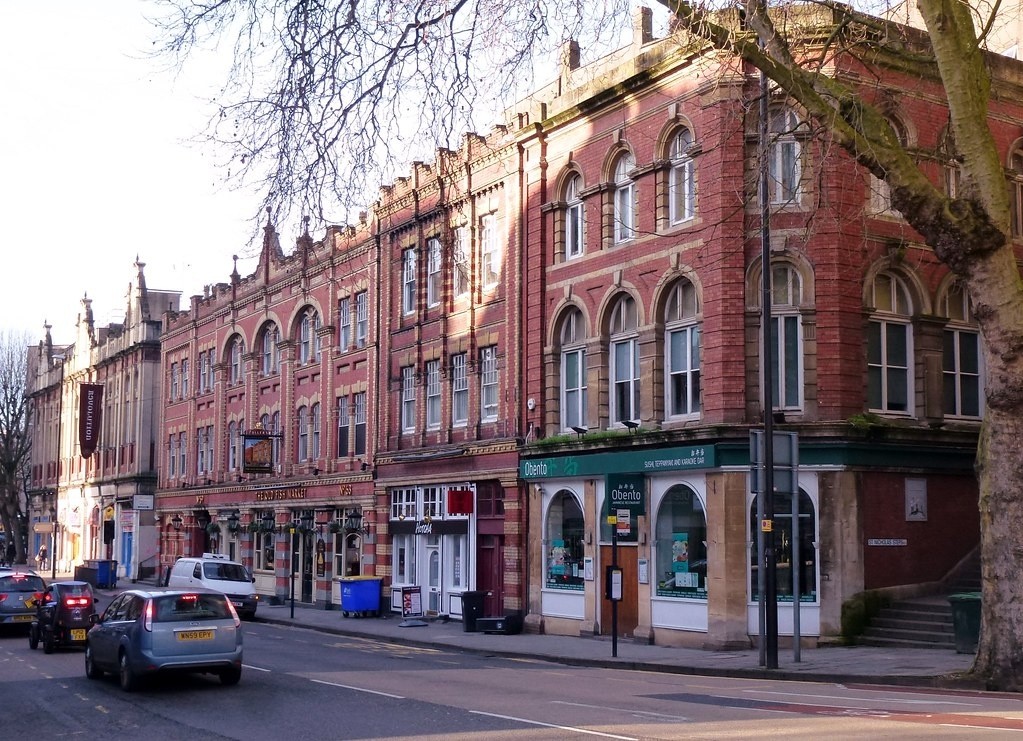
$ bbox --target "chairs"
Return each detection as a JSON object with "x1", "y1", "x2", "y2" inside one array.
[{"x1": 175, "y1": 599, "x2": 194, "y2": 610}]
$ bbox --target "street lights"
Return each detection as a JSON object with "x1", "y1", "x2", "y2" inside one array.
[
  {"x1": 290, "y1": 523, "x2": 297, "y2": 618},
  {"x1": 49, "y1": 506, "x2": 57, "y2": 579}
]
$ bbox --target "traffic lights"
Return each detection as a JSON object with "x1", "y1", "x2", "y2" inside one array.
[{"x1": 104, "y1": 520, "x2": 111, "y2": 544}]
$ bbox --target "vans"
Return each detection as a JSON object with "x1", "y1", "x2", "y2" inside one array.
[{"x1": 168, "y1": 552, "x2": 258, "y2": 620}]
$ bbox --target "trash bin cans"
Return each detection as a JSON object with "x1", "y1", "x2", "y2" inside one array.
[
  {"x1": 459, "y1": 591, "x2": 484, "y2": 632},
  {"x1": 83, "y1": 559, "x2": 118, "y2": 588},
  {"x1": 948, "y1": 592, "x2": 982, "y2": 654},
  {"x1": 341, "y1": 575, "x2": 382, "y2": 618}
]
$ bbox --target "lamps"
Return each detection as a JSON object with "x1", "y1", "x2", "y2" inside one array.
[
  {"x1": 262, "y1": 512, "x2": 281, "y2": 534},
  {"x1": 229, "y1": 513, "x2": 246, "y2": 534},
  {"x1": 197, "y1": 513, "x2": 211, "y2": 529},
  {"x1": 348, "y1": 507, "x2": 369, "y2": 538},
  {"x1": 300, "y1": 512, "x2": 327, "y2": 534},
  {"x1": 173, "y1": 516, "x2": 182, "y2": 531}
]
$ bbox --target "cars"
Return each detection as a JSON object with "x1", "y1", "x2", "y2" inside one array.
[
  {"x1": 0, "y1": 571, "x2": 47, "y2": 624},
  {"x1": 28, "y1": 581, "x2": 99, "y2": 654},
  {"x1": 85, "y1": 586, "x2": 244, "y2": 692}
]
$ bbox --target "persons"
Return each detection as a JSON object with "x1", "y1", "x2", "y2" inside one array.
[{"x1": 38, "y1": 544, "x2": 47, "y2": 571}]
